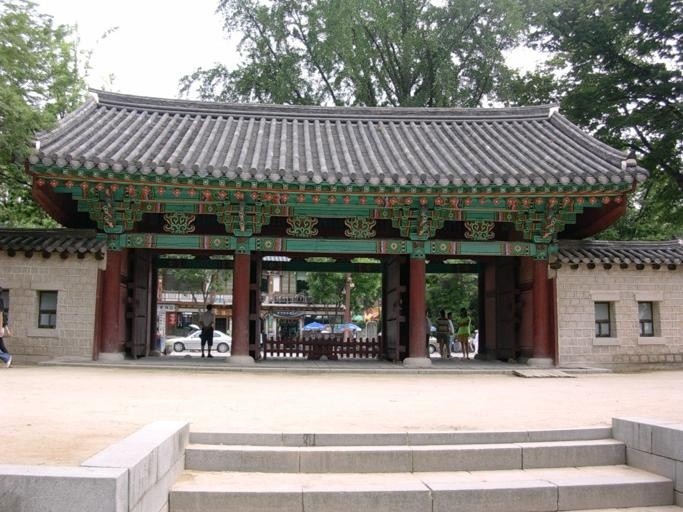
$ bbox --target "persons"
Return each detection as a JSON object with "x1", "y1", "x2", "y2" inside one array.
[
  {"x1": 0, "y1": 308, "x2": 11, "y2": 353},
  {"x1": 435, "y1": 309, "x2": 453, "y2": 360},
  {"x1": 456, "y1": 307, "x2": 470, "y2": 360},
  {"x1": 445, "y1": 311, "x2": 454, "y2": 358},
  {"x1": 0, "y1": 349, "x2": 11, "y2": 369},
  {"x1": 197, "y1": 302, "x2": 214, "y2": 358},
  {"x1": 424, "y1": 310, "x2": 430, "y2": 349}
]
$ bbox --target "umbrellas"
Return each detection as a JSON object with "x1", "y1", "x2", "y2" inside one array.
[{"x1": 301, "y1": 321, "x2": 362, "y2": 335}]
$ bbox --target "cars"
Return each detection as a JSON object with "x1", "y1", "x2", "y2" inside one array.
[
  {"x1": 165, "y1": 330, "x2": 232, "y2": 353},
  {"x1": 428, "y1": 336, "x2": 439, "y2": 354}
]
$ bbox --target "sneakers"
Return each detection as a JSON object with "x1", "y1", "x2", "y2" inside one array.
[
  {"x1": 201, "y1": 354, "x2": 213, "y2": 358},
  {"x1": 440, "y1": 354, "x2": 471, "y2": 360},
  {"x1": 6, "y1": 355, "x2": 13, "y2": 367}
]
